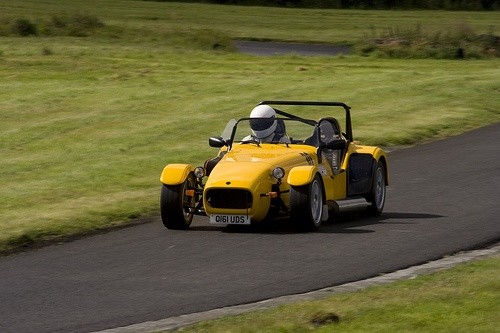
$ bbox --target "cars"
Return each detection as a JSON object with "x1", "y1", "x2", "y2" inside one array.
[{"x1": 159, "y1": 100, "x2": 391, "y2": 230}]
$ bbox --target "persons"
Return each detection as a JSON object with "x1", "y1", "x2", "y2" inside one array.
[{"x1": 238, "y1": 103, "x2": 291, "y2": 143}]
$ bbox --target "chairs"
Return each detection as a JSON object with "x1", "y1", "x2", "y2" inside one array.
[
  {"x1": 274, "y1": 119, "x2": 286, "y2": 135},
  {"x1": 304, "y1": 117, "x2": 347, "y2": 175}
]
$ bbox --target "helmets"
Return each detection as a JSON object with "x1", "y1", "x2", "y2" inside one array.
[{"x1": 249, "y1": 105, "x2": 277, "y2": 139}]
